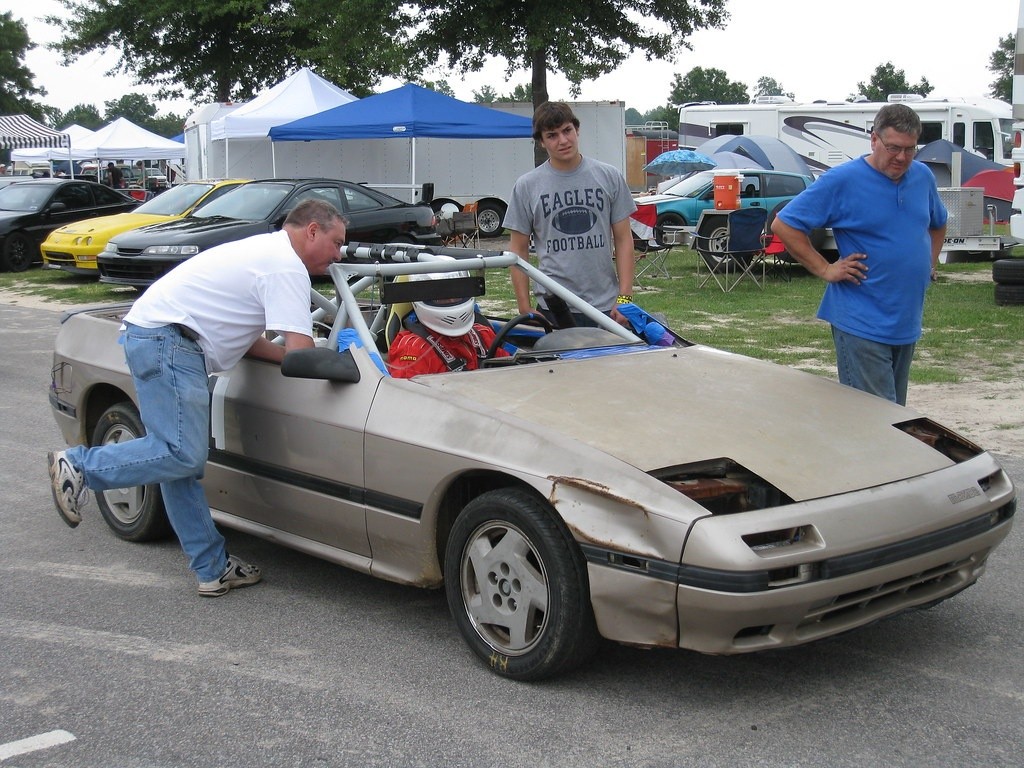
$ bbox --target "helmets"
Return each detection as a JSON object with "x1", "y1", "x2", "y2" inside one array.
[{"x1": 408, "y1": 255, "x2": 476, "y2": 335}]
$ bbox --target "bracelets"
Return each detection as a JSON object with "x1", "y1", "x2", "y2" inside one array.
[
  {"x1": 932, "y1": 267, "x2": 934, "y2": 270},
  {"x1": 616, "y1": 294, "x2": 633, "y2": 304}
]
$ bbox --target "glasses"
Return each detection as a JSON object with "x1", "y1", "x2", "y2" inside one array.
[{"x1": 876, "y1": 133, "x2": 919, "y2": 155}]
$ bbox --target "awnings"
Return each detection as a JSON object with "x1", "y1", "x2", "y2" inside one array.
[
  {"x1": 0, "y1": 112, "x2": 74, "y2": 179},
  {"x1": 73, "y1": 117, "x2": 185, "y2": 189},
  {"x1": 171, "y1": 67, "x2": 533, "y2": 206},
  {"x1": 11, "y1": 124, "x2": 95, "y2": 180}
]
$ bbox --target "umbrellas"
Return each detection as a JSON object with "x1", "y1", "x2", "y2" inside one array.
[{"x1": 642, "y1": 135, "x2": 815, "y2": 181}]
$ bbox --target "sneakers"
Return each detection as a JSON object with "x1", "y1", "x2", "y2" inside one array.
[
  {"x1": 48, "y1": 451, "x2": 90, "y2": 528},
  {"x1": 199, "y1": 554, "x2": 264, "y2": 597}
]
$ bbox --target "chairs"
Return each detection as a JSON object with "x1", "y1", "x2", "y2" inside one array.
[
  {"x1": 745, "y1": 184, "x2": 756, "y2": 198},
  {"x1": 630, "y1": 204, "x2": 684, "y2": 291},
  {"x1": 752, "y1": 233, "x2": 789, "y2": 282},
  {"x1": 384, "y1": 275, "x2": 416, "y2": 351},
  {"x1": 689, "y1": 208, "x2": 770, "y2": 292}
]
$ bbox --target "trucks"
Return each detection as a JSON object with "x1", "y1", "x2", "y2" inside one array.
[
  {"x1": 12, "y1": 161, "x2": 54, "y2": 176},
  {"x1": 676, "y1": 93, "x2": 1015, "y2": 166}
]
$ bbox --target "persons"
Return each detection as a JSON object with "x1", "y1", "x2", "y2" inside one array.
[
  {"x1": 108, "y1": 162, "x2": 125, "y2": 189},
  {"x1": 136, "y1": 161, "x2": 149, "y2": 185},
  {"x1": 771, "y1": 104, "x2": 948, "y2": 406},
  {"x1": 502, "y1": 102, "x2": 638, "y2": 327},
  {"x1": 54, "y1": 168, "x2": 68, "y2": 177},
  {"x1": 47, "y1": 199, "x2": 345, "y2": 597},
  {"x1": 388, "y1": 256, "x2": 510, "y2": 380},
  {"x1": 43, "y1": 170, "x2": 50, "y2": 178},
  {"x1": 0, "y1": 163, "x2": 9, "y2": 177}
]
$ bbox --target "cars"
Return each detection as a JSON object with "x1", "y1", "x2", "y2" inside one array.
[
  {"x1": 80, "y1": 165, "x2": 168, "y2": 190},
  {"x1": 95, "y1": 179, "x2": 439, "y2": 293},
  {"x1": 630, "y1": 167, "x2": 815, "y2": 247},
  {"x1": 40, "y1": 181, "x2": 291, "y2": 273},
  {"x1": 0, "y1": 178, "x2": 148, "y2": 273},
  {"x1": 48, "y1": 248, "x2": 1015, "y2": 683},
  {"x1": 0, "y1": 176, "x2": 34, "y2": 202}
]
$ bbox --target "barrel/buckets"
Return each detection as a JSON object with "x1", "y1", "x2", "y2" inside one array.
[{"x1": 713, "y1": 169, "x2": 742, "y2": 211}]
